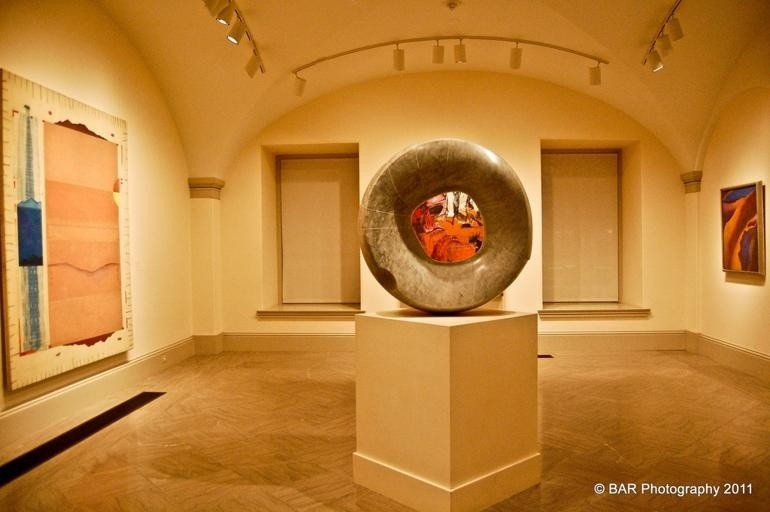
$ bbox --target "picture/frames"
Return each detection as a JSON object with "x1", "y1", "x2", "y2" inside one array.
[{"x1": 721, "y1": 180, "x2": 766, "y2": 276}]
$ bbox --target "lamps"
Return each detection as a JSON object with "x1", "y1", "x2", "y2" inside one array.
[
  {"x1": 208, "y1": 0, "x2": 266, "y2": 78},
  {"x1": 292, "y1": 36, "x2": 610, "y2": 97},
  {"x1": 642, "y1": 0, "x2": 686, "y2": 73}
]
